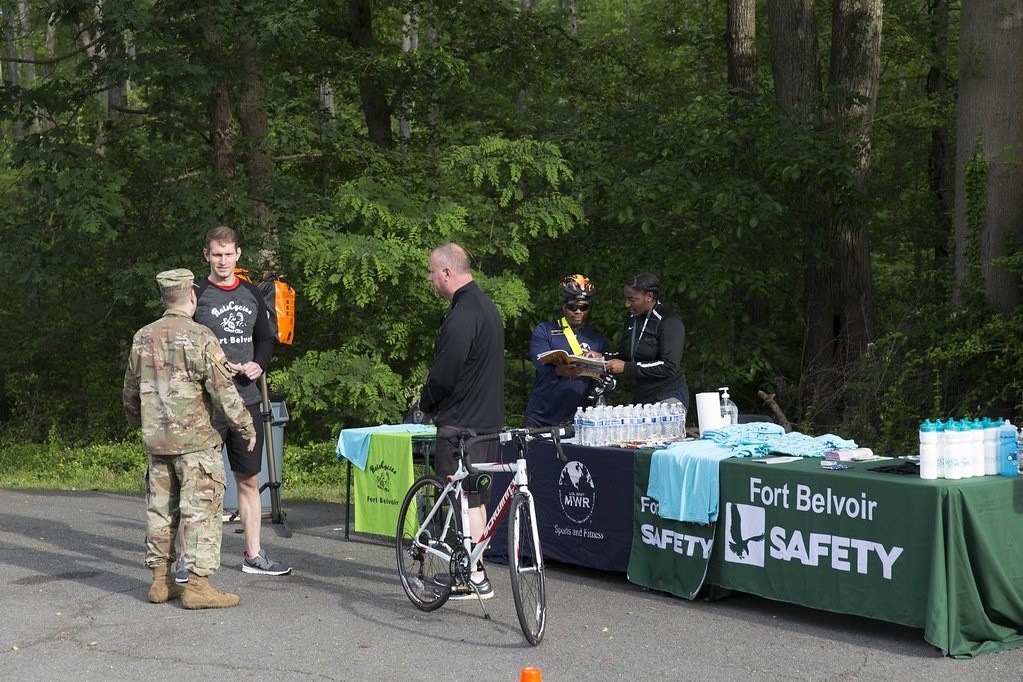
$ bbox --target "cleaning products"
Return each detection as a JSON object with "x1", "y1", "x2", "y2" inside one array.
[{"x1": 919, "y1": 417, "x2": 1019, "y2": 480}]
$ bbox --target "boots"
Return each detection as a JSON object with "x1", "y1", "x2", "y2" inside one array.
[
  {"x1": 148, "y1": 563, "x2": 182, "y2": 603},
  {"x1": 181, "y1": 570, "x2": 239, "y2": 609}
]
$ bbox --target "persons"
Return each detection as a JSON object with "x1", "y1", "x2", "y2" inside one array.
[
  {"x1": 579, "y1": 270, "x2": 689, "y2": 411},
  {"x1": 175, "y1": 227, "x2": 292, "y2": 582},
  {"x1": 520, "y1": 274, "x2": 618, "y2": 428},
  {"x1": 417, "y1": 243, "x2": 504, "y2": 599},
  {"x1": 123, "y1": 268, "x2": 257, "y2": 610}
]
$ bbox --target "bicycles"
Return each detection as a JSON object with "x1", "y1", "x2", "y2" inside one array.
[{"x1": 395, "y1": 425, "x2": 569, "y2": 646}]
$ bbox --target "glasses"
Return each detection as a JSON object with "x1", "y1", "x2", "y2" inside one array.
[{"x1": 565, "y1": 303, "x2": 591, "y2": 312}]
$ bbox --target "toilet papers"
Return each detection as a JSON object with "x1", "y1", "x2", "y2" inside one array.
[{"x1": 695, "y1": 393, "x2": 722, "y2": 441}]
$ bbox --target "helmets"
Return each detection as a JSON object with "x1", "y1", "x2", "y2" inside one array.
[{"x1": 559, "y1": 274, "x2": 596, "y2": 299}]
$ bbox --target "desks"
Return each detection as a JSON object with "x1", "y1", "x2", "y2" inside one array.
[{"x1": 333, "y1": 422, "x2": 1023, "y2": 658}]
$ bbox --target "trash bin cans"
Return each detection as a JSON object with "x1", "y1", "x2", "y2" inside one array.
[{"x1": 219, "y1": 388, "x2": 294, "y2": 521}]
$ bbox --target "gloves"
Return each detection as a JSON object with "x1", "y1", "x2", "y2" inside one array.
[{"x1": 587, "y1": 374, "x2": 617, "y2": 398}]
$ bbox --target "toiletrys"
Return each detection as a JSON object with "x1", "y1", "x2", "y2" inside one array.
[{"x1": 718, "y1": 387, "x2": 738, "y2": 428}]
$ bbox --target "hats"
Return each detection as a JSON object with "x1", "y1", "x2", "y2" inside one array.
[{"x1": 156, "y1": 268, "x2": 200, "y2": 293}]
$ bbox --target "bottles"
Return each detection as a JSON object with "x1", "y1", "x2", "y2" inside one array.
[
  {"x1": 574, "y1": 402, "x2": 686, "y2": 447},
  {"x1": 919, "y1": 417, "x2": 1023, "y2": 479}
]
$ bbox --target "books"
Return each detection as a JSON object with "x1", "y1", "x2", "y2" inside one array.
[{"x1": 536, "y1": 350, "x2": 606, "y2": 377}]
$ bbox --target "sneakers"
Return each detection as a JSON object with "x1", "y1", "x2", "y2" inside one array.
[
  {"x1": 433, "y1": 577, "x2": 495, "y2": 600},
  {"x1": 432, "y1": 573, "x2": 458, "y2": 587},
  {"x1": 174, "y1": 561, "x2": 190, "y2": 581},
  {"x1": 241, "y1": 548, "x2": 292, "y2": 575}
]
